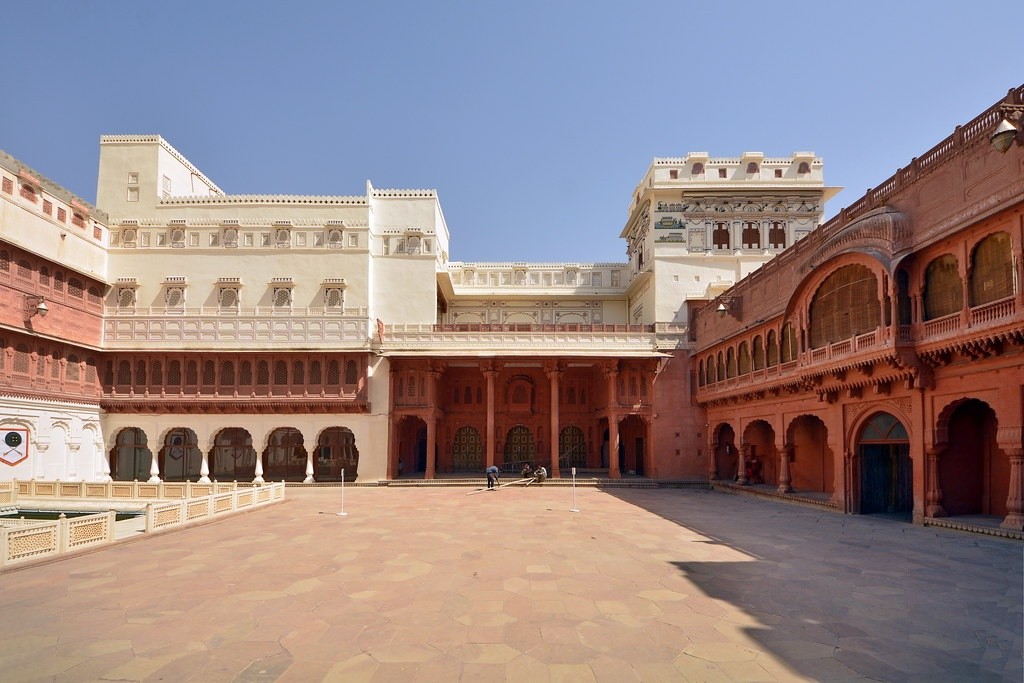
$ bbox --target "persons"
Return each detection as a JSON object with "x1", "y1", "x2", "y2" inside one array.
[
  {"x1": 485, "y1": 466, "x2": 502, "y2": 490},
  {"x1": 521, "y1": 464, "x2": 533, "y2": 477},
  {"x1": 534, "y1": 463, "x2": 547, "y2": 483}
]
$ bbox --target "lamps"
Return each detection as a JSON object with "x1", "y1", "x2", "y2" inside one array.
[
  {"x1": 717, "y1": 295, "x2": 743, "y2": 320},
  {"x1": 726, "y1": 446, "x2": 731, "y2": 456},
  {"x1": 989, "y1": 103, "x2": 1024, "y2": 155},
  {"x1": 24, "y1": 295, "x2": 48, "y2": 322}
]
